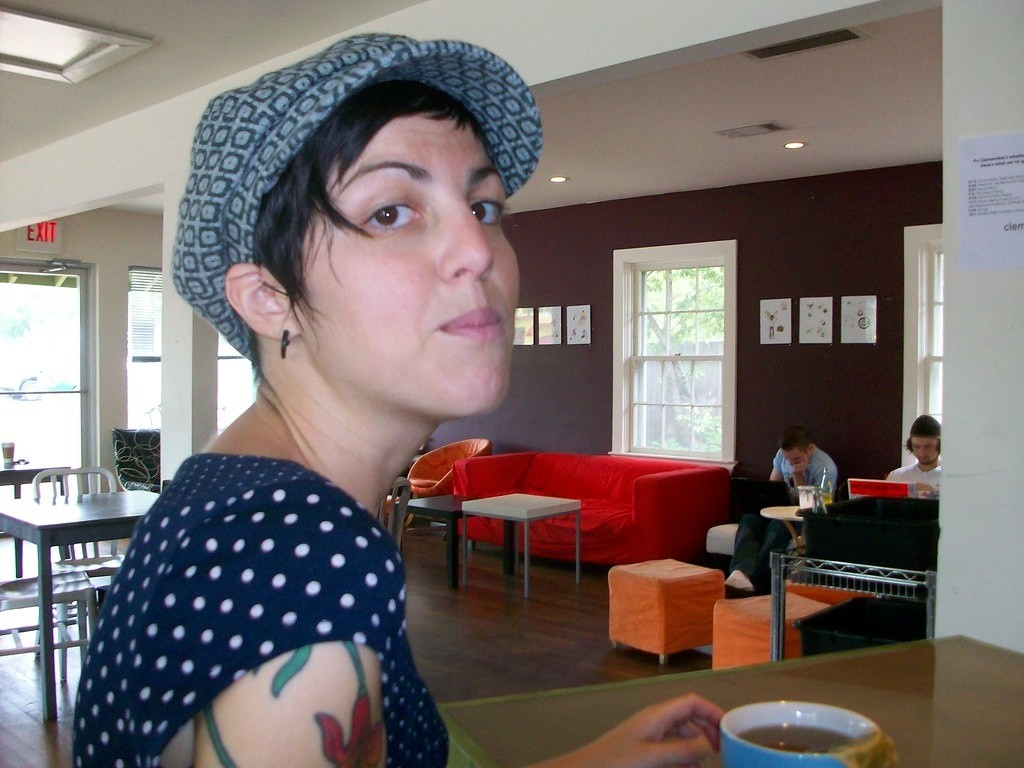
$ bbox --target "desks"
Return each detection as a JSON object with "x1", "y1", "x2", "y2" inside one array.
[
  {"x1": 0, "y1": 467, "x2": 72, "y2": 578},
  {"x1": 760, "y1": 505, "x2": 806, "y2": 567},
  {"x1": 433, "y1": 634, "x2": 1024, "y2": 768},
  {"x1": 0, "y1": 489, "x2": 162, "y2": 722}
]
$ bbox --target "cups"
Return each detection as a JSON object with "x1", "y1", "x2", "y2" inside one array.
[
  {"x1": 797, "y1": 485, "x2": 818, "y2": 509},
  {"x1": 2, "y1": 443, "x2": 15, "y2": 464},
  {"x1": 718, "y1": 700, "x2": 881, "y2": 768}
]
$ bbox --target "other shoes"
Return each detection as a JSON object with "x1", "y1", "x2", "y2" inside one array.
[{"x1": 723, "y1": 582, "x2": 755, "y2": 596}]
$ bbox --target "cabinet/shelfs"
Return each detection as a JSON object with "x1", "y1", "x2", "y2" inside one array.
[{"x1": 767, "y1": 550, "x2": 935, "y2": 656}]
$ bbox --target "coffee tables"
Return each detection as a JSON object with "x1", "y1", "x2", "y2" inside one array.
[
  {"x1": 393, "y1": 495, "x2": 516, "y2": 590},
  {"x1": 461, "y1": 493, "x2": 582, "y2": 599}
]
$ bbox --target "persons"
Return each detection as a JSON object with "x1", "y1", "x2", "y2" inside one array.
[
  {"x1": 72, "y1": 32, "x2": 725, "y2": 768},
  {"x1": 725, "y1": 425, "x2": 838, "y2": 599},
  {"x1": 885, "y1": 415, "x2": 941, "y2": 499}
]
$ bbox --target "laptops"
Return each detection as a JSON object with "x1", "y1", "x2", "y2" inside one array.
[{"x1": 730, "y1": 476, "x2": 792, "y2": 515}]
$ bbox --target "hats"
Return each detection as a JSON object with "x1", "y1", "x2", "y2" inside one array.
[
  {"x1": 171, "y1": 32, "x2": 542, "y2": 361},
  {"x1": 910, "y1": 415, "x2": 941, "y2": 436}
]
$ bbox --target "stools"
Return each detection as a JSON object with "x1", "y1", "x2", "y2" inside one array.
[
  {"x1": 789, "y1": 597, "x2": 927, "y2": 657},
  {"x1": 705, "y1": 523, "x2": 739, "y2": 556},
  {"x1": 785, "y1": 579, "x2": 876, "y2": 605},
  {"x1": 711, "y1": 591, "x2": 832, "y2": 670},
  {"x1": 607, "y1": 559, "x2": 726, "y2": 667}
]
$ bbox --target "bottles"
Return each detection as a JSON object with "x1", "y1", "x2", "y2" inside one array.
[{"x1": 819, "y1": 466, "x2": 833, "y2": 505}]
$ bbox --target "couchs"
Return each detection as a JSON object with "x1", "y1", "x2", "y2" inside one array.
[{"x1": 452, "y1": 451, "x2": 732, "y2": 576}]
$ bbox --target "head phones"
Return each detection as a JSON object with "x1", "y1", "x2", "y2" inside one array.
[{"x1": 907, "y1": 437, "x2": 941, "y2": 454}]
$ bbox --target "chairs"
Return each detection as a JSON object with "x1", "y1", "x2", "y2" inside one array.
[
  {"x1": 0, "y1": 466, "x2": 127, "y2": 684},
  {"x1": 112, "y1": 428, "x2": 160, "y2": 494},
  {"x1": 384, "y1": 439, "x2": 494, "y2": 543},
  {"x1": 378, "y1": 477, "x2": 411, "y2": 552}
]
